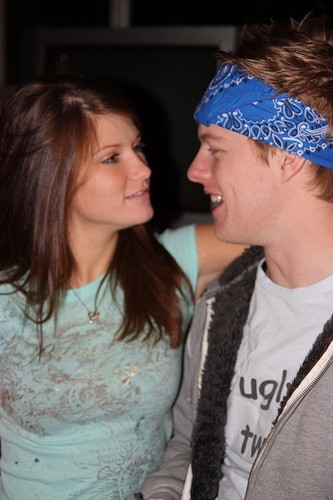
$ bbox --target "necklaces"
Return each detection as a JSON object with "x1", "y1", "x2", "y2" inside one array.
[{"x1": 59, "y1": 275, "x2": 112, "y2": 324}]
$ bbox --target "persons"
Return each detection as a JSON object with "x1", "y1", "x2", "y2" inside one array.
[
  {"x1": 1, "y1": 78, "x2": 253, "y2": 500},
  {"x1": 137, "y1": 14, "x2": 333, "y2": 500}
]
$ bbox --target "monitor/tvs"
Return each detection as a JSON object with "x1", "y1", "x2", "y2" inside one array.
[{"x1": 19, "y1": 19, "x2": 242, "y2": 214}]
